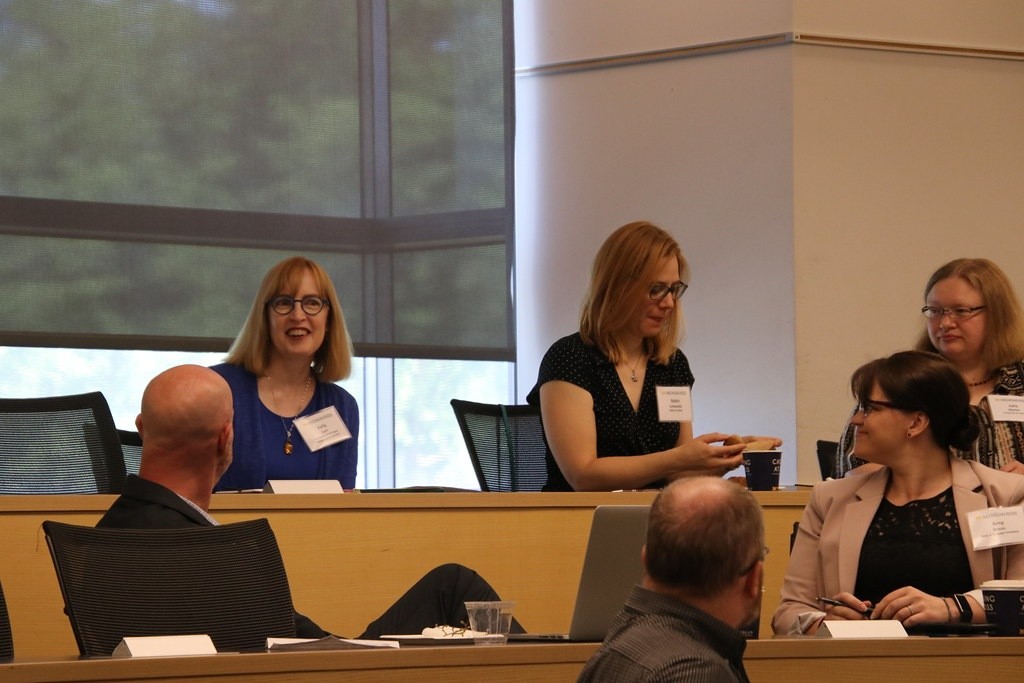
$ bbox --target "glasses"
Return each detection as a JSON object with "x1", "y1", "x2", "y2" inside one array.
[
  {"x1": 922, "y1": 304, "x2": 987, "y2": 321},
  {"x1": 267, "y1": 295, "x2": 330, "y2": 315},
  {"x1": 859, "y1": 400, "x2": 893, "y2": 418},
  {"x1": 649, "y1": 281, "x2": 688, "y2": 301}
]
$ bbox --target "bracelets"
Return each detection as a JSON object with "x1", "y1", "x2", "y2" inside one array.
[{"x1": 942, "y1": 597, "x2": 952, "y2": 623}]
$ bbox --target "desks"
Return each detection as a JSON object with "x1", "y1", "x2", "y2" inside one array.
[
  {"x1": 0, "y1": 636, "x2": 1024, "y2": 683},
  {"x1": 0, "y1": 484, "x2": 811, "y2": 660}
]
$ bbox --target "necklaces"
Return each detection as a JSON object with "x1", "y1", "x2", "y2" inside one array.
[
  {"x1": 267, "y1": 375, "x2": 311, "y2": 455},
  {"x1": 626, "y1": 354, "x2": 642, "y2": 382},
  {"x1": 967, "y1": 378, "x2": 992, "y2": 387}
]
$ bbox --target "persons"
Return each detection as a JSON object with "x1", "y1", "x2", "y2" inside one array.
[
  {"x1": 93, "y1": 366, "x2": 526, "y2": 640},
  {"x1": 525, "y1": 221, "x2": 782, "y2": 493},
  {"x1": 771, "y1": 350, "x2": 1024, "y2": 637},
  {"x1": 571, "y1": 476, "x2": 766, "y2": 683},
  {"x1": 209, "y1": 255, "x2": 359, "y2": 495},
  {"x1": 831, "y1": 257, "x2": 1024, "y2": 473}
]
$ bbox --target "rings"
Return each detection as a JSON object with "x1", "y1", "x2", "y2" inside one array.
[{"x1": 908, "y1": 606, "x2": 913, "y2": 615}]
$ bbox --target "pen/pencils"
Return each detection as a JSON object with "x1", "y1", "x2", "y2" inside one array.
[{"x1": 816, "y1": 597, "x2": 873, "y2": 615}]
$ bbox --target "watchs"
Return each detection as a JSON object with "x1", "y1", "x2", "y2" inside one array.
[{"x1": 953, "y1": 593, "x2": 973, "y2": 623}]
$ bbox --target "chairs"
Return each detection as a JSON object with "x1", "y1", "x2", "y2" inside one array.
[
  {"x1": 0, "y1": 581, "x2": 15, "y2": 664},
  {"x1": 451, "y1": 398, "x2": 559, "y2": 492},
  {"x1": 81, "y1": 420, "x2": 144, "y2": 493},
  {"x1": 816, "y1": 439, "x2": 839, "y2": 481},
  {"x1": 41, "y1": 518, "x2": 296, "y2": 656},
  {"x1": 0, "y1": 391, "x2": 128, "y2": 494}
]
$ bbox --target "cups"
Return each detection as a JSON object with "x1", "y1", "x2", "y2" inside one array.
[
  {"x1": 742, "y1": 451, "x2": 782, "y2": 492},
  {"x1": 980, "y1": 580, "x2": 1024, "y2": 637},
  {"x1": 465, "y1": 602, "x2": 515, "y2": 644}
]
